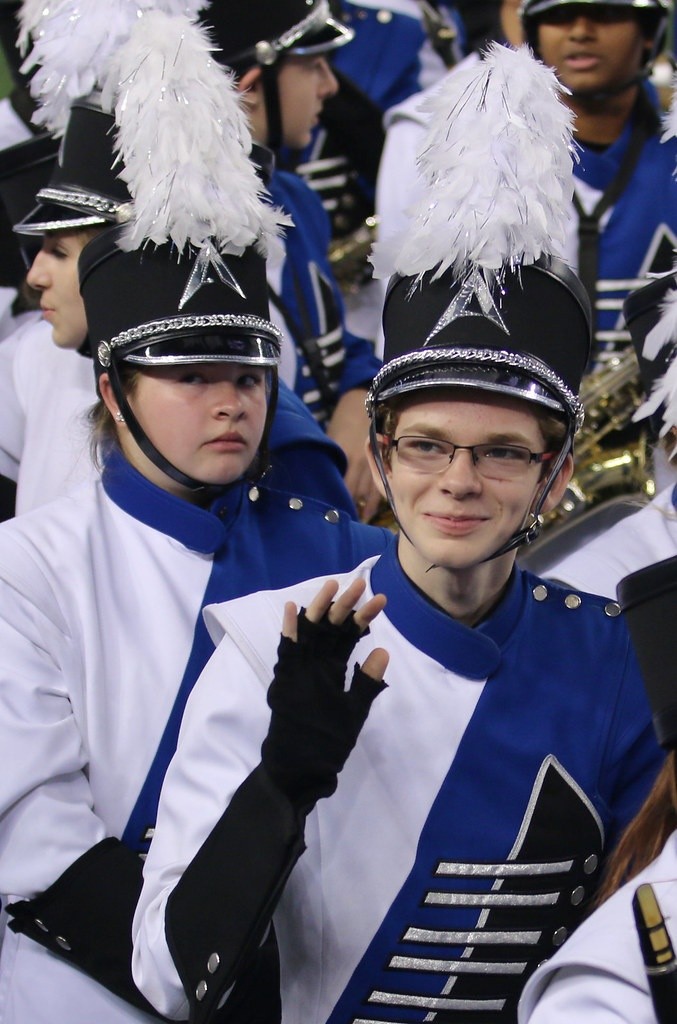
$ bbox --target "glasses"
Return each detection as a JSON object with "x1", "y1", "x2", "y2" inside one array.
[{"x1": 382, "y1": 436, "x2": 561, "y2": 481}]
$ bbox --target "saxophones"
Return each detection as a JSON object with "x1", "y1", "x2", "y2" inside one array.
[
  {"x1": 534, "y1": 345, "x2": 657, "y2": 534},
  {"x1": 327, "y1": 211, "x2": 379, "y2": 300}
]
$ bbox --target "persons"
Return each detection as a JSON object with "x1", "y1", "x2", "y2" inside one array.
[
  {"x1": 536, "y1": 273, "x2": 677, "y2": 597},
  {"x1": 12, "y1": 94, "x2": 358, "y2": 522},
  {"x1": 132, "y1": 305, "x2": 663, "y2": 1024},
  {"x1": 319, "y1": 0, "x2": 468, "y2": 222},
  {"x1": 518, "y1": 651, "x2": 677, "y2": 1024},
  {"x1": 1, "y1": 271, "x2": 391, "y2": 1024},
  {"x1": 0, "y1": 0, "x2": 49, "y2": 152},
  {"x1": 520, "y1": 0, "x2": 677, "y2": 370},
  {"x1": 0, "y1": 133, "x2": 62, "y2": 338},
  {"x1": 197, "y1": 1, "x2": 384, "y2": 524}
]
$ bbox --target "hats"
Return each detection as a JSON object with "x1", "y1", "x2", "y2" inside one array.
[
  {"x1": 13, "y1": 0, "x2": 275, "y2": 240},
  {"x1": 75, "y1": 11, "x2": 283, "y2": 398},
  {"x1": 364, "y1": 40, "x2": 594, "y2": 417},
  {"x1": 195, "y1": 0, "x2": 357, "y2": 71},
  {"x1": 623, "y1": 269, "x2": 677, "y2": 436},
  {"x1": 520, "y1": 0, "x2": 669, "y2": 30}
]
